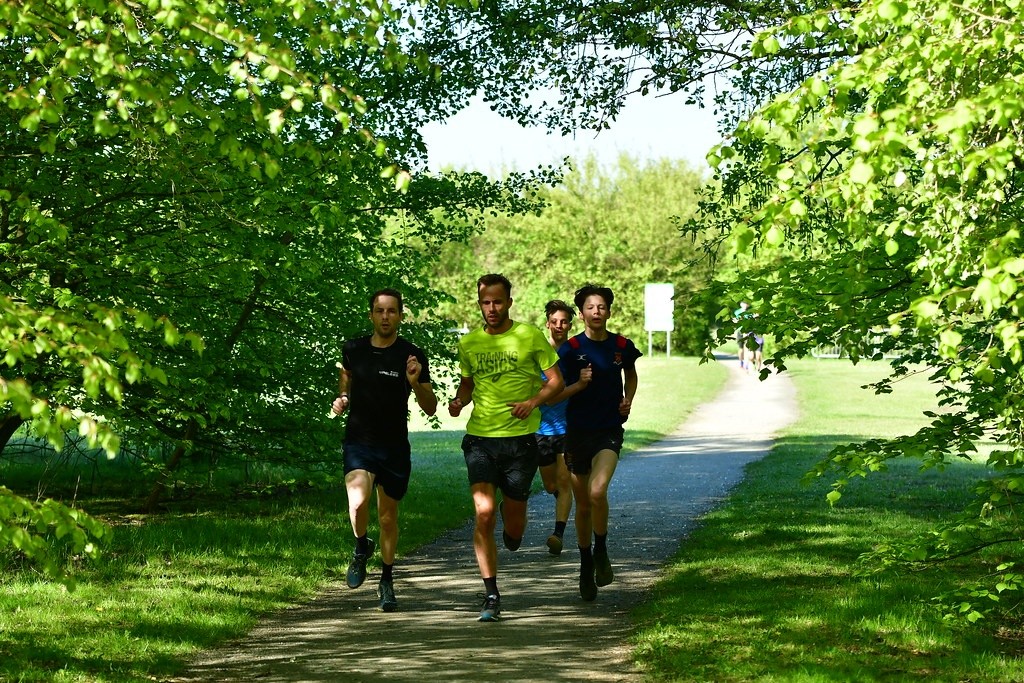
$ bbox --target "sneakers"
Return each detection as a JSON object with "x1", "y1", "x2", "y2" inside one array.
[
  {"x1": 593, "y1": 549, "x2": 615, "y2": 587},
  {"x1": 377, "y1": 580, "x2": 398, "y2": 612},
  {"x1": 500, "y1": 500, "x2": 522, "y2": 552},
  {"x1": 347, "y1": 538, "x2": 375, "y2": 588},
  {"x1": 476, "y1": 592, "x2": 500, "y2": 622},
  {"x1": 580, "y1": 565, "x2": 597, "y2": 603}
]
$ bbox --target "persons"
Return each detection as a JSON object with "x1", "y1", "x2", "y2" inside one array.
[
  {"x1": 732, "y1": 300, "x2": 763, "y2": 372},
  {"x1": 559, "y1": 285, "x2": 642, "y2": 601},
  {"x1": 333, "y1": 289, "x2": 438, "y2": 608},
  {"x1": 449, "y1": 274, "x2": 565, "y2": 622},
  {"x1": 538, "y1": 300, "x2": 575, "y2": 554}
]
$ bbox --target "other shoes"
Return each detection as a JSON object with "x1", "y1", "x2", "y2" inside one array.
[
  {"x1": 546, "y1": 535, "x2": 563, "y2": 555},
  {"x1": 553, "y1": 491, "x2": 557, "y2": 499}
]
